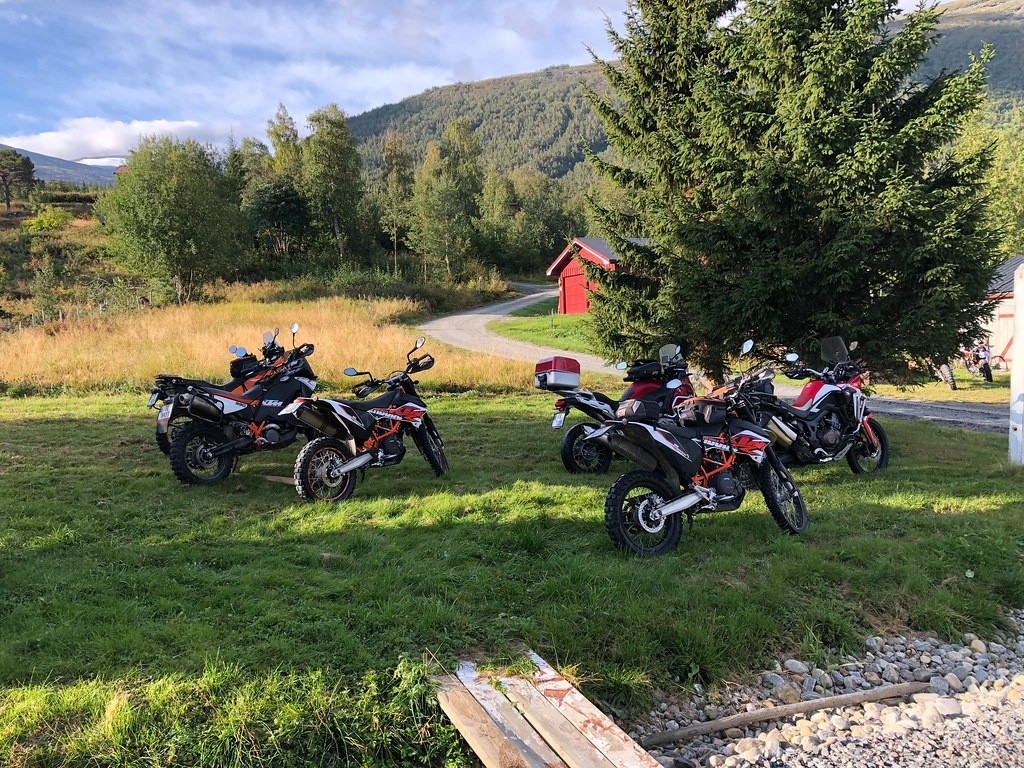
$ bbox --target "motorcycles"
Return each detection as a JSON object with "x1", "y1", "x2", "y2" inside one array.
[
  {"x1": 534, "y1": 335, "x2": 890, "y2": 559},
  {"x1": 964, "y1": 337, "x2": 993, "y2": 382},
  {"x1": 146, "y1": 322, "x2": 320, "y2": 486},
  {"x1": 924, "y1": 350, "x2": 958, "y2": 391},
  {"x1": 277, "y1": 336, "x2": 450, "y2": 507}
]
